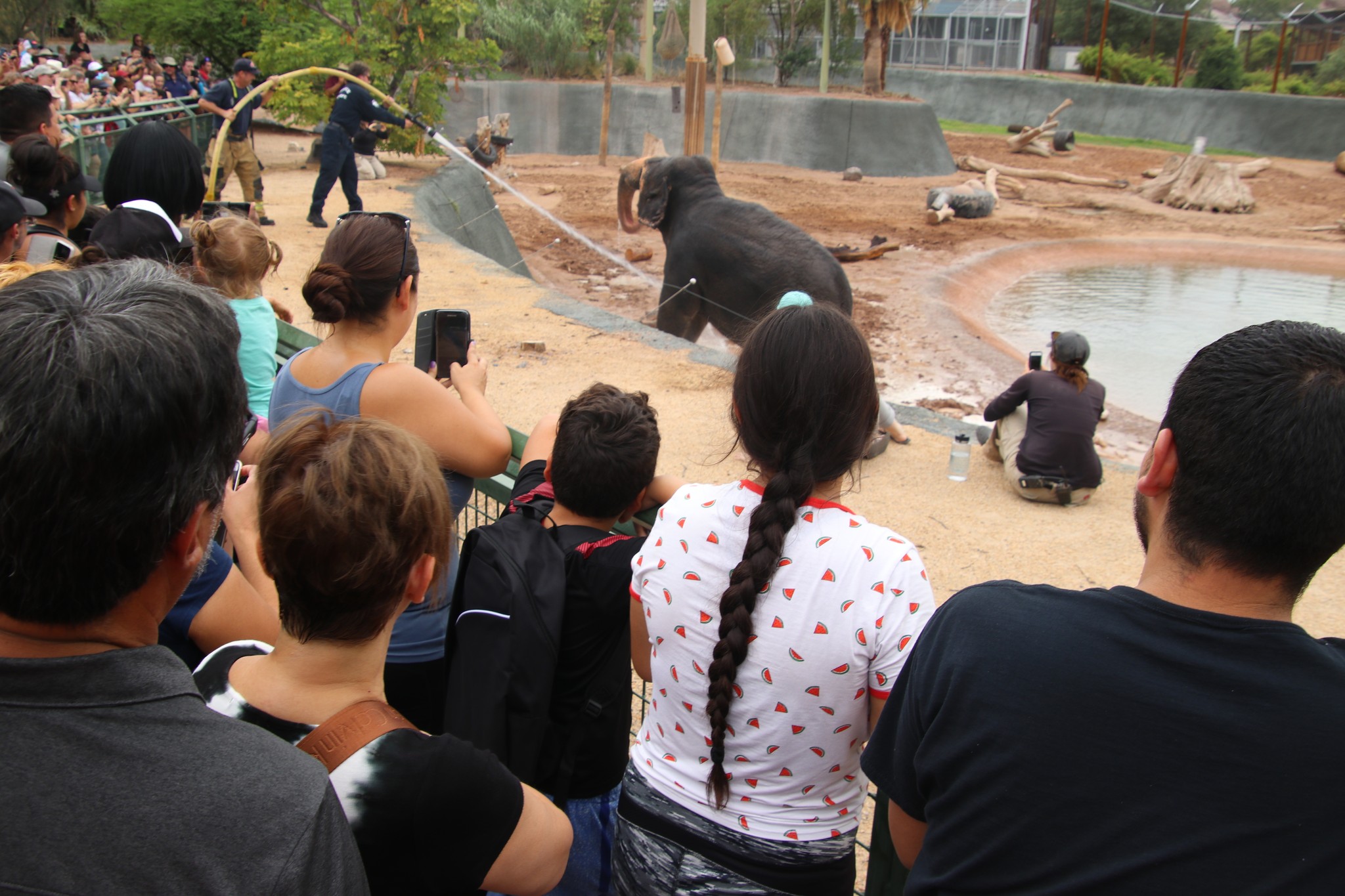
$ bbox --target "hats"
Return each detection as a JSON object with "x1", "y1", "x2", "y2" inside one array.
[
  {"x1": 20, "y1": 38, "x2": 44, "y2": 49},
  {"x1": 30, "y1": 65, "x2": 59, "y2": 78},
  {"x1": 32, "y1": 48, "x2": 59, "y2": 62},
  {"x1": 88, "y1": 61, "x2": 103, "y2": 72},
  {"x1": 1046, "y1": 331, "x2": 1089, "y2": 366},
  {"x1": 161, "y1": 56, "x2": 179, "y2": 67},
  {"x1": 0, "y1": 181, "x2": 47, "y2": 232},
  {"x1": 44, "y1": 59, "x2": 69, "y2": 74},
  {"x1": 233, "y1": 59, "x2": 261, "y2": 74},
  {"x1": 21, "y1": 171, "x2": 103, "y2": 201}
]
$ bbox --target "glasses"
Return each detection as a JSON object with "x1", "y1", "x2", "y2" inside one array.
[
  {"x1": 334, "y1": 210, "x2": 412, "y2": 297},
  {"x1": 363, "y1": 73, "x2": 371, "y2": 81}
]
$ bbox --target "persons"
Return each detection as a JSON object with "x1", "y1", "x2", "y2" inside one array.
[
  {"x1": 610, "y1": 300, "x2": 936, "y2": 896},
  {"x1": 303, "y1": 60, "x2": 412, "y2": 231},
  {"x1": 0, "y1": 179, "x2": 49, "y2": 286},
  {"x1": 984, "y1": 328, "x2": 1106, "y2": 506},
  {"x1": 187, "y1": 214, "x2": 281, "y2": 427},
  {"x1": 82, "y1": 197, "x2": 290, "y2": 465},
  {"x1": 352, "y1": 94, "x2": 396, "y2": 179},
  {"x1": 443, "y1": 383, "x2": 696, "y2": 896},
  {"x1": 162, "y1": 449, "x2": 288, "y2": 704},
  {"x1": 856, "y1": 319, "x2": 1345, "y2": 896},
  {"x1": 771, "y1": 285, "x2": 908, "y2": 461},
  {"x1": 0, "y1": 11, "x2": 300, "y2": 324},
  {"x1": 177, "y1": 402, "x2": 577, "y2": 895},
  {"x1": 268, "y1": 205, "x2": 513, "y2": 747},
  {"x1": 1, "y1": 247, "x2": 376, "y2": 895},
  {"x1": 196, "y1": 60, "x2": 278, "y2": 233}
]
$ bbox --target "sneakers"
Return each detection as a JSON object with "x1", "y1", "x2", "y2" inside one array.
[{"x1": 307, "y1": 211, "x2": 327, "y2": 228}]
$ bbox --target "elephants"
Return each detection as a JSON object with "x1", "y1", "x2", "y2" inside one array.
[{"x1": 617, "y1": 155, "x2": 852, "y2": 343}]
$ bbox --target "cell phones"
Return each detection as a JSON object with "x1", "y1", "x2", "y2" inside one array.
[
  {"x1": 53, "y1": 240, "x2": 74, "y2": 265},
  {"x1": 435, "y1": 309, "x2": 471, "y2": 379},
  {"x1": 1029, "y1": 352, "x2": 1042, "y2": 371},
  {"x1": 202, "y1": 201, "x2": 251, "y2": 222}
]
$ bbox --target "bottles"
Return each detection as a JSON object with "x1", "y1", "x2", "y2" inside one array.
[{"x1": 945, "y1": 434, "x2": 971, "y2": 482}]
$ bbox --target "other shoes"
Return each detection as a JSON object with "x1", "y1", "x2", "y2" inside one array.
[{"x1": 259, "y1": 216, "x2": 275, "y2": 225}]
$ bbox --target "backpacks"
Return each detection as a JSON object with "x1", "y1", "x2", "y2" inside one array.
[{"x1": 438, "y1": 500, "x2": 619, "y2": 793}]
